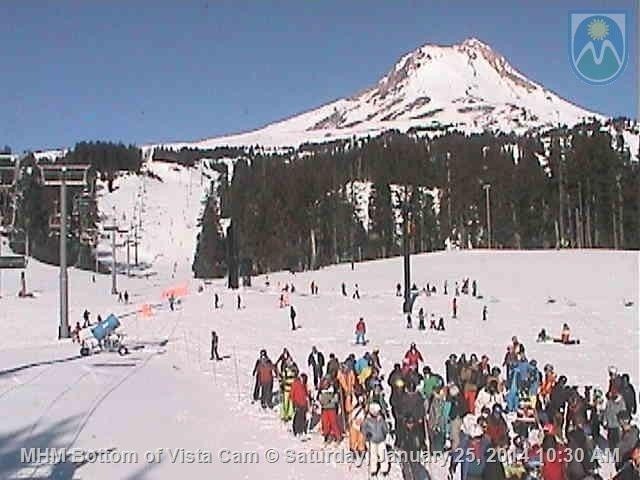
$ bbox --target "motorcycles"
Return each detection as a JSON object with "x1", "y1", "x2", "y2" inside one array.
[{"x1": 78, "y1": 322, "x2": 128, "y2": 356}]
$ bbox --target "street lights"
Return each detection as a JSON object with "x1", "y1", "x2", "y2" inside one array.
[
  {"x1": 482, "y1": 185, "x2": 493, "y2": 250},
  {"x1": 40, "y1": 165, "x2": 96, "y2": 337}
]
